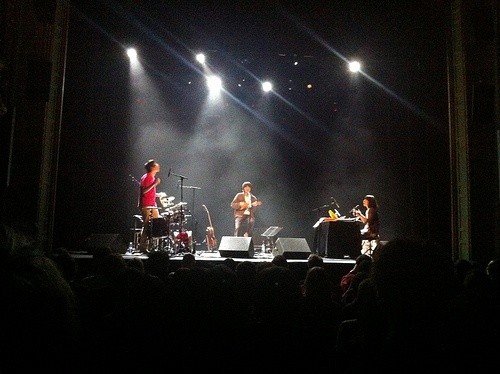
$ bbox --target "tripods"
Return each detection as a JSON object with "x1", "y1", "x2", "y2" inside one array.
[{"x1": 154, "y1": 173, "x2": 188, "y2": 254}]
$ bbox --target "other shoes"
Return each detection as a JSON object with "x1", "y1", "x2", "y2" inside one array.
[{"x1": 141, "y1": 249, "x2": 151, "y2": 254}]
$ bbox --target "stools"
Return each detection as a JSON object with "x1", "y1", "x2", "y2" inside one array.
[
  {"x1": 131, "y1": 228, "x2": 143, "y2": 254},
  {"x1": 362, "y1": 236, "x2": 381, "y2": 255}
]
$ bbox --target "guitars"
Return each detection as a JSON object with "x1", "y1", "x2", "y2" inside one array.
[{"x1": 201, "y1": 204, "x2": 216, "y2": 250}]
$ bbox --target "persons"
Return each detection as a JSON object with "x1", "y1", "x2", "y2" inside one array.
[
  {"x1": 356, "y1": 194, "x2": 379, "y2": 239},
  {"x1": 138, "y1": 160, "x2": 160, "y2": 253},
  {"x1": 0, "y1": 225, "x2": 500, "y2": 374},
  {"x1": 231, "y1": 181, "x2": 258, "y2": 236}
]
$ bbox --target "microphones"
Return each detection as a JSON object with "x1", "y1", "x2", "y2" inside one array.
[
  {"x1": 168, "y1": 168, "x2": 171, "y2": 177},
  {"x1": 177, "y1": 180, "x2": 180, "y2": 189},
  {"x1": 331, "y1": 197, "x2": 339, "y2": 208},
  {"x1": 349, "y1": 205, "x2": 359, "y2": 214}
]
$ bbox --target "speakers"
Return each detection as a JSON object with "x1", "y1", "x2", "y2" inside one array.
[
  {"x1": 272, "y1": 238, "x2": 311, "y2": 259},
  {"x1": 86, "y1": 233, "x2": 128, "y2": 255},
  {"x1": 219, "y1": 235, "x2": 254, "y2": 258}
]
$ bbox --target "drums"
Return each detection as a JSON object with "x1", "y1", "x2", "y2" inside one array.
[
  {"x1": 147, "y1": 218, "x2": 168, "y2": 239},
  {"x1": 165, "y1": 211, "x2": 186, "y2": 225},
  {"x1": 163, "y1": 231, "x2": 193, "y2": 254}
]
